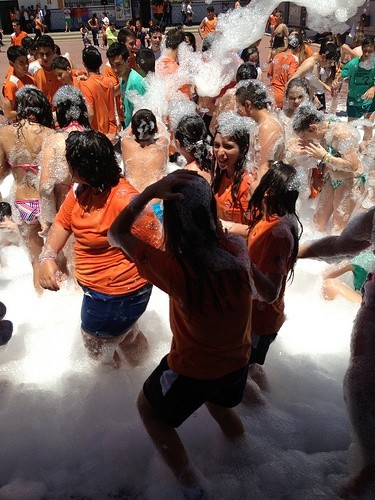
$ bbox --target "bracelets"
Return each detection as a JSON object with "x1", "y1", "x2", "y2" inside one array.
[
  {"x1": 38, "y1": 253, "x2": 57, "y2": 260},
  {"x1": 325, "y1": 154, "x2": 330, "y2": 163}
]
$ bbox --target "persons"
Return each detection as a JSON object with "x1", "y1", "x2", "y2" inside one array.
[
  {"x1": 38, "y1": 84, "x2": 91, "y2": 293},
  {"x1": 0, "y1": 84, "x2": 57, "y2": 295},
  {"x1": 119, "y1": 107, "x2": 168, "y2": 226},
  {"x1": 211, "y1": 116, "x2": 251, "y2": 225},
  {"x1": 0, "y1": 0, "x2": 375, "y2": 189},
  {"x1": 297, "y1": 206, "x2": 375, "y2": 258},
  {"x1": 361, "y1": 136, "x2": 375, "y2": 203},
  {"x1": 234, "y1": 79, "x2": 285, "y2": 189},
  {"x1": 107, "y1": 170, "x2": 252, "y2": 490},
  {"x1": 340, "y1": 265, "x2": 375, "y2": 500},
  {"x1": 292, "y1": 102, "x2": 365, "y2": 233},
  {"x1": 0, "y1": 202, "x2": 20, "y2": 247},
  {"x1": 38, "y1": 129, "x2": 165, "y2": 369},
  {"x1": 323, "y1": 249, "x2": 375, "y2": 302},
  {"x1": 334, "y1": 35, "x2": 375, "y2": 142},
  {"x1": 244, "y1": 160, "x2": 303, "y2": 407},
  {"x1": 174, "y1": 113, "x2": 213, "y2": 184},
  {"x1": 0, "y1": 301, "x2": 13, "y2": 346}
]
